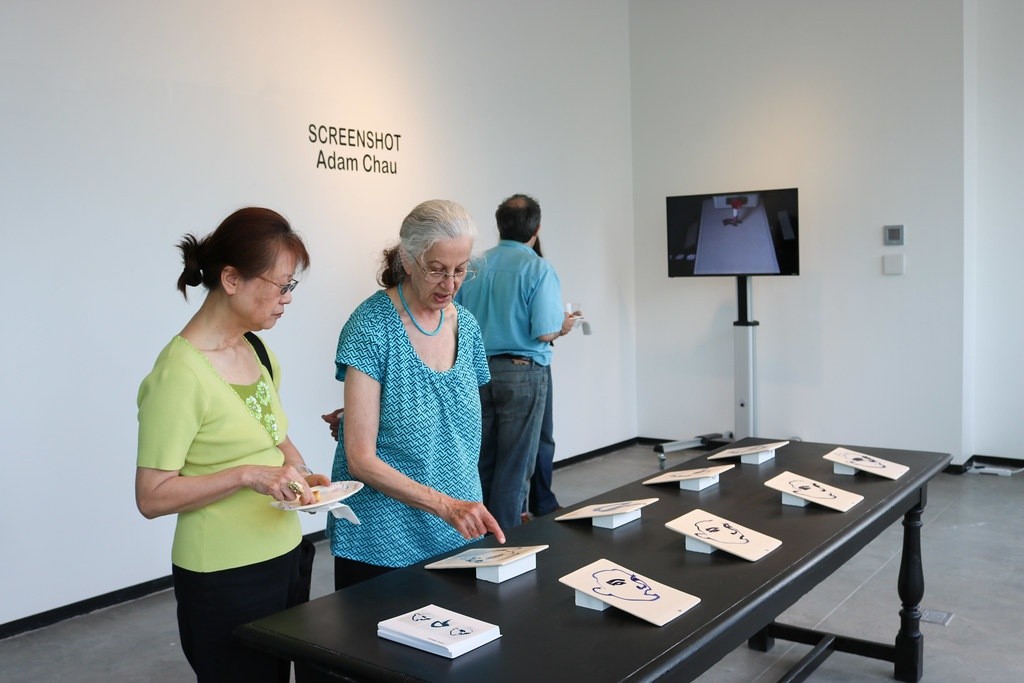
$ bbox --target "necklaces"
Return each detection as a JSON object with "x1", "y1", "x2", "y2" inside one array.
[{"x1": 398, "y1": 280, "x2": 445, "y2": 336}]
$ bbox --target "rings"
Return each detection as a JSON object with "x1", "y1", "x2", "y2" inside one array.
[{"x1": 287, "y1": 480, "x2": 304, "y2": 494}]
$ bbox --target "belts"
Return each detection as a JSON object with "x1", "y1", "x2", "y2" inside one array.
[{"x1": 490, "y1": 354, "x2": 531, "y2": 363}]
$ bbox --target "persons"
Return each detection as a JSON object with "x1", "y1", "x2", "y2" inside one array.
[
  {"x1": 322, "y1": 199, "x2": 507, "y2": 591},
  {"x1": 451, "y1": 194, "x2": 575, "y2": 539},
  {"x1": 521, "y1": 237, "x2": 565, "y2": 520},
  {"x1": 134, "y1": 207, "x2": 330, "y2": 683}
]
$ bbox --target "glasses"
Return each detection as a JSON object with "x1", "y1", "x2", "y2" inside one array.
[
  {"x1": 252, "y1": 275, "x2": 298, "y2": 295},
  {"x1": 408, "y1": 250, "x2": 477, "y2": 285}
]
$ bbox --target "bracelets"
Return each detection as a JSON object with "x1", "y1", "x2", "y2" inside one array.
[{"x1": 558, "y1": 330, "x2": 568, "y2": 336}]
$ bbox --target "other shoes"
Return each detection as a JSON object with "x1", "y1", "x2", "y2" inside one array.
[{"x1": 522, "y1": 516, "x2": 529, "y2": 523}]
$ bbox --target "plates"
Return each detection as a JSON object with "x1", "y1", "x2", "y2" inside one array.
[
  {"x1": 283, "y1": 480, "x2": 364, "y2": 512},
  {"x1": 565, "y1": 315, "x2": 585, "y2": 321}
]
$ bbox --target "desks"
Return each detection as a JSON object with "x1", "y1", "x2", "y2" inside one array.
[{"x1": 238, "y1": 437, "x2": 953, "y2": 683}]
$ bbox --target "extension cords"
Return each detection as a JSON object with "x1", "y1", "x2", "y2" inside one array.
[{"x1": 968, "y1": 467, "x2": 1012, "y2": 476}]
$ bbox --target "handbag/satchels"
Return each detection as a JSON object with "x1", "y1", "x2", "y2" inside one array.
[{"x1": 298, "y1": 539, "x2": 315, "y2": 603}]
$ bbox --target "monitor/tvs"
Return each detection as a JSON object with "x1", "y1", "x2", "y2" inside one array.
[{"x1": 666, "y1": 188, "x2": 800, "y2": 278}]
